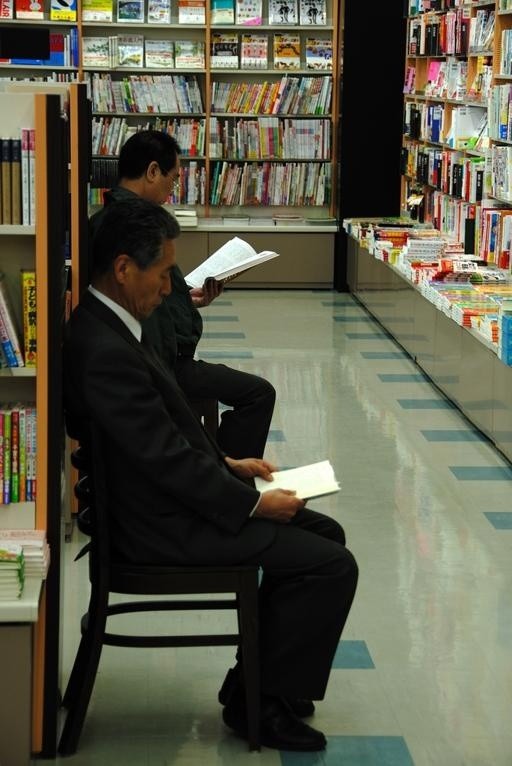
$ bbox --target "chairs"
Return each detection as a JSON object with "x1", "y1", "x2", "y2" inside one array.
[{"x1": 51, "y1": 356, "x2": 272, "y2": 757}]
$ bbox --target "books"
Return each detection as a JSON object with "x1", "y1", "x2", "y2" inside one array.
[
  {"x1": 181, "y1": 236, "x2": 280, "y2": 290},
  {"x1": 342, "y1": 1, "x2": 512, "y2": 363},
  {"x1": 82, "y1": 0, "x2": 337, "y2": 230},
  {"x1": 253, "y1": 459, "x2": 342, "y2": 503},
  {"x1": 1, "y1": 0, "x2": 78, "y2": 606}
]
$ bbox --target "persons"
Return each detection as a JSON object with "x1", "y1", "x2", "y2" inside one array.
[
  {"x1": 85, "y1": 132, "x2": 276, "y2": 461},
  {"x1": 62, "y1": 197, "x2": 359, "y2": 751}
]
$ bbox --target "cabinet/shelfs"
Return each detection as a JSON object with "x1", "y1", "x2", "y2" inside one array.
[
  {"x1": 0, "y1": 2, "x2": 343, "y2": 294},
  {"x1": 2, "y1": 82, "x2": 95, "y2": 766},
  {"x1": 344, "y1": 7, "x2": 511, "y2": 469}
]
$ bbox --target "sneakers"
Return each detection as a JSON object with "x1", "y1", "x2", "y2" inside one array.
[
  {"x1": 223, "y1": 706, "x2": 326, "y2": 752},
  {"x1": 219, "y1": 669, "x2": 316, "y2": 717}
]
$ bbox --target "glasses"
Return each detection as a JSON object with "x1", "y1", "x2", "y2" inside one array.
[{"x1": 164, "y1": 169, "x2": 180, "y2": 189}]
